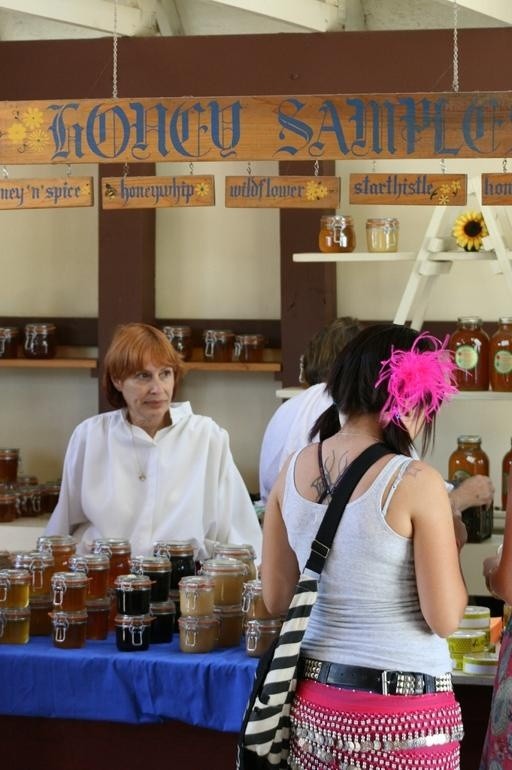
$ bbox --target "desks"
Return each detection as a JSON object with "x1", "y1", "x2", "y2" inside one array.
[{"x1": 0, "y1": 626, "x2": 494, "y2": 770}]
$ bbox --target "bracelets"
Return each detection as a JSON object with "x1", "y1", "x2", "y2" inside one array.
[{"x1": 486, "y1": 566, "x2": 502, "y2": 599}]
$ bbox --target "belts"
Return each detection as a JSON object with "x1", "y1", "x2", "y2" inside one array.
[{"x1": 302, "y1": 657, "x2": 453, "y2": 695}]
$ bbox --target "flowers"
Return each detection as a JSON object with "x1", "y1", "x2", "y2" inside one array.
[{"x1": 452, "y1": 210, "x2": 489, "y2": 250}]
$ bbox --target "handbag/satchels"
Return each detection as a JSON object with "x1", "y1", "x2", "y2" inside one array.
[{"x1": 234, "y1": 568, "x2": 320, "y2": 770}]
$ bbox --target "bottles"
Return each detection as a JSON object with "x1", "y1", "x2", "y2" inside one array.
[{"x1": 503, "y1": 440, "x2": 512, "y2": 511}]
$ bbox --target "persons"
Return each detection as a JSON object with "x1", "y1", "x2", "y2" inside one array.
[
  {"x1": 481, "y1": 464, "x2": 511, "y2": 770},
  {"x1": 257, "y1": 315, "x2": 495, "y2": 520},
  {"x1": 37, "y1": 321, "x2": 263, "y2": 582},
  {"x1": 258, "y1": 319, "x2": 469, "y2": 769}
]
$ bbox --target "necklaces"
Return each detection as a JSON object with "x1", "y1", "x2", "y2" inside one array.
[
  {"x1": 333, "y1": 431, "x2": 385, "y2": 443},
  {"x1": 126, "y1": 409, "x2": 161, "y2": 481}
]
